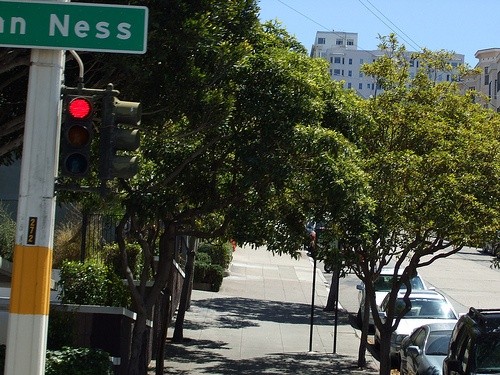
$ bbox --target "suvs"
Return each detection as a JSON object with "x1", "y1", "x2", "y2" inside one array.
[{"x1": 441, "y1": 307, "x2": 500, "y2": 375}]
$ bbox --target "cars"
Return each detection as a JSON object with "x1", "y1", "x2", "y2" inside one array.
[
  {"x1": 306, "y1": 218, "x2": 325, "y2": 241},
  {"x1": 398, "y1": 322, "x2": 456, "y2": 375},
  {"x1": 356, "y1": 266, "x2": 435, "y2": 333},
  {"x1": 373, "y1": 290, "x2": 458, "y2": 364}
]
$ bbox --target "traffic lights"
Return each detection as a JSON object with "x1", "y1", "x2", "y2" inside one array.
[
  {"x1": 59, "y1": 94, "x2": 97, "y2": 179},
  {"x1": 97, "y1": 82, "x2": 144, "y2": 183}
]
